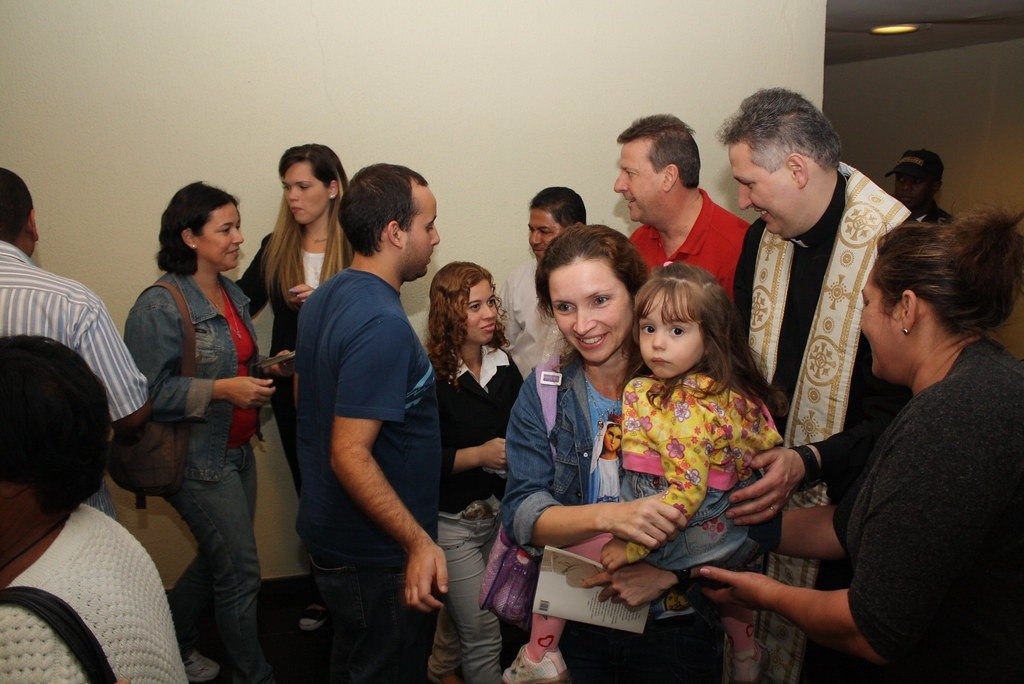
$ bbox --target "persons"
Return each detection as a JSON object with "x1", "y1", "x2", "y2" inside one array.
[
  {"x1": 885, "y1": 149, "x2": 956, "y2": 223},
  {"x1": 495, "y1": 186, "x2": 586, "y2": 381},
  {"x1": 699, "y1": 194, "x2": 1024, "y2": 684},
  {"x1": 499, "y1": 223, "x2": 789, "y2": 684},
  {"x1": 293, "y1": 162, "x2": 524, "y2": 684},
  {"x1": 716, "y1": 87, "x2": 923, "y2": 684},
  {"x1": 0, "y1": 168, "x2": 193, "y2": 684},
  {"x1": 124, "y1": 143, "x2": 350, "y2": 684},
  {"x1": 613, "y1": 111, "x2": 750, "y2": 305}
]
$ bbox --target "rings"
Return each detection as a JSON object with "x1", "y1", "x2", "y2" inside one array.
[{"x1": 770, "y1": 507, "x2": 777, "y2": 514}]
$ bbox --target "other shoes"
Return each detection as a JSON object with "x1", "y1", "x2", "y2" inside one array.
[
  {"x1": 428, "y1": 670, "x2": 462, "y2": 684},
  {"x1": 298, "y1": 599, "x2": 330, "y2": 630}
]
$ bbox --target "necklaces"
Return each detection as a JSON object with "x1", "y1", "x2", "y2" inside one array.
[{"x1": 199, "y1": 282, "x2": 242, "y2": 339}]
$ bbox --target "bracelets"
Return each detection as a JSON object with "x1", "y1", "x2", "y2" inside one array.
[{"x1": 789, "y1": 443, "x2": 820, "y2": 492}]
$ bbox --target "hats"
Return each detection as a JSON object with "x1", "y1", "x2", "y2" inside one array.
[{"x1": 884, "y1": 149, "x2": 945, "y2": 184}]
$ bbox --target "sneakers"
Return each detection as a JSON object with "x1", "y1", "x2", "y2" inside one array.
[
  {"x1": 501, "y1": 639, "x2": 568, "y2": 684},
  {"x1": 731, "y1": 640, "x2": 770, "y2": 684},
  {"x1": 182, "y1": 651, "x2": 219, "y2": 683}
]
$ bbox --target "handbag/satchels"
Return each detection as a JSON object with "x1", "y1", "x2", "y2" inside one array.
[
  {"x1": 478, "y1": 521, "x2": 542, "y2": 633},
  {"x1": 106, "y1": 285, "x2": 201, "y2": 513}
]
